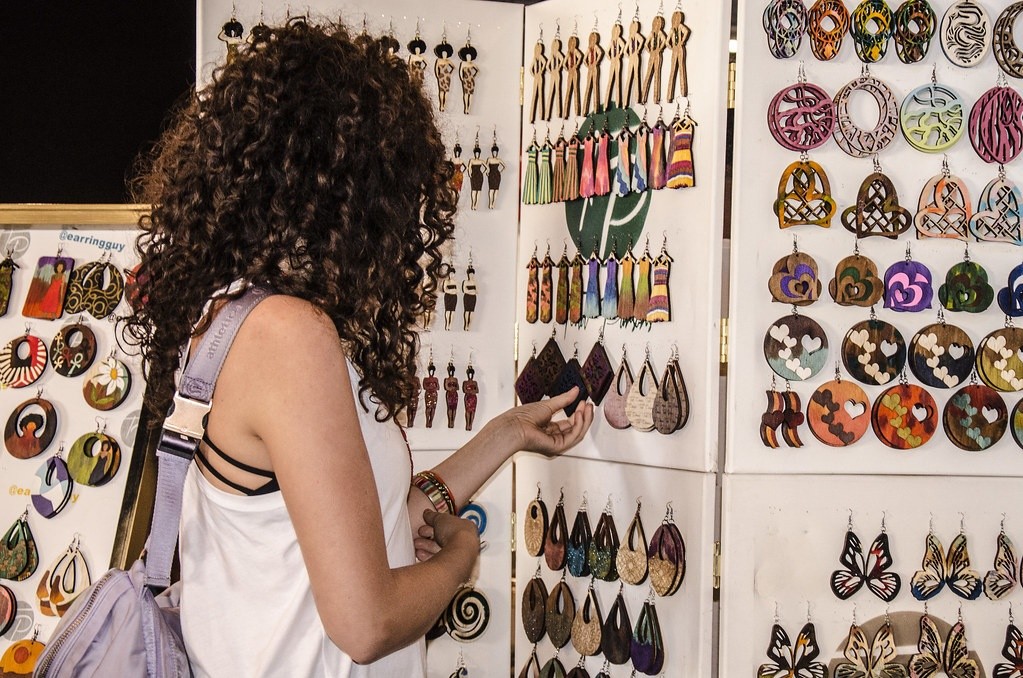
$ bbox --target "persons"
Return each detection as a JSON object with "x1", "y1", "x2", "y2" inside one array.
[
  {"x1": 434, "y1": 42, "x2": 455, "y2": 112},
  {"x1": 218, "y1": 18, "x2": 246, "y2": 67},
  {"x1": 486, "y1": 144, "x2": 505, "y2": 209},
  {"x1": 423, "y1": 363, "x2": 440, "y2": 428},
  {"x1": 468, "y1": 146, "x2": 488, "y2": 211},
  {"x1": 405, "y1": 364, "x2": 422, "y2": 428},
  {"x1": 354, "y1": 34, "x2": 373, "y2": 53},
  {"x1": 462, "y1": 366, "x2": 479, "y2": 431},
  {"x1": 458, "y1": 44, "x2": 480, "y2": 115},
  {"x1": 463, "y1": 266, "x2": 477, "y2": 332},
  {"x1": 449, "y1": 144, "x2": 466, "y2": 208},
  {"x1": 247, "y1": 21, "x2": 271, "y2": 52},
  {"x1": 407, "y1": 39, "x2": 428, "y2": 87},
  {"x1": 116, "y1": 20, "x2": 595, "y2": 678},
  {"x1": 443, "y1": 362, "x2": 459, "y2": 428},
  {"x1": 422, "y1": 266, "x2": 459, "y2": 332},
  {"x1": 380, "y1": 37, "x2": 403, "y2": 67}
]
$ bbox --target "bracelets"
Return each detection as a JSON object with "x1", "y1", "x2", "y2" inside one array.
[{"x1": 411, "y1": 470, "x2": 458, "y2": 517}]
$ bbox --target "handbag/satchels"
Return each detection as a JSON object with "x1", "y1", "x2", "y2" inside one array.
[{"x1": 30, "y1": 534, "x2": 192, "y2": 678}]
